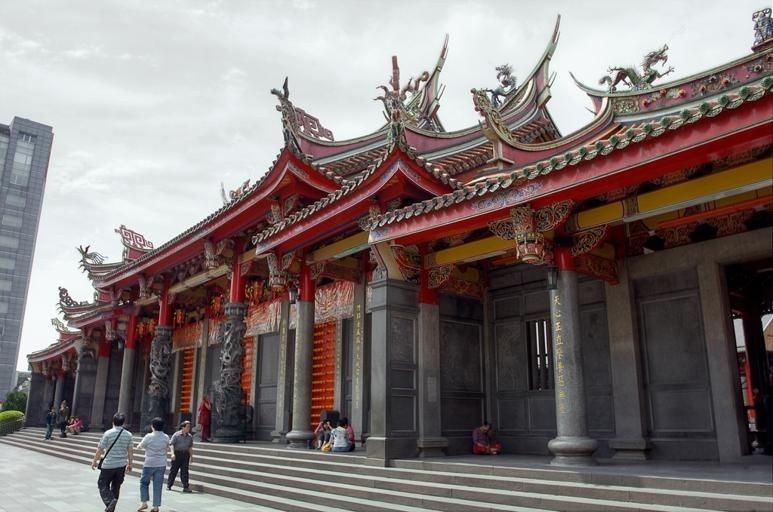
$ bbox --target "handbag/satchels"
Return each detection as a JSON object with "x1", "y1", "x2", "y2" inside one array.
[
  {"x1": 97, "y1": 458, "x2": 102, "y2": 469},
  {"x1": 321, "y1": 443, "x2": 330, "y2": 451}
]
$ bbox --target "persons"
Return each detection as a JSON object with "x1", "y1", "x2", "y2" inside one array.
[
  {"x1": 91, "y1": 412, "x2": 133, "y2": 512},
  {"x1": 136, "y1": 416, "x2": 169, "y2": 512},
  {"x1": 166, "y1": 420, "x2": 194, "y2": 493},
  {"x1": 198, "y1": 394, "x2": 213, "y2": 440},
  {"x1": 43, "y1": 400, "x2": 89, "y2": 440},
  {"x1": 471, "y1": 424, "x2": 505, "y2": 456},
  {"x1": 313, "y1": 417, "x2": 355, "y2": 452}
]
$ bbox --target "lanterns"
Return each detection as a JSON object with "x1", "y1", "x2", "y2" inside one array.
[
  {"x1": 211, "y1": 295, "x2": 222, "y2": 319},
  {"x1": 174, "y1": 306, "x2": 185, "y2": 328},
  {"x1": 136, "y1": 315, "x2": 156, "y2": 339},
  {"x1": 251, "y1": 278, "x2": 266, "y2": 308}
]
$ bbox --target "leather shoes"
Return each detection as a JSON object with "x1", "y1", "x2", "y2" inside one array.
[{"x1": 104, "y1": 498, "x2": 117, "y2": 512}]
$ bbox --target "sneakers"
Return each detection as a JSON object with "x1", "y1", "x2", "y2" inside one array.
[{"x1": 183, "y1": 489, "x2": 191, "y2": 493}]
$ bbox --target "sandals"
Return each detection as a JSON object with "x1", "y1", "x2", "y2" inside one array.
[
  {"x1": 151, "y1": 508, "x2": 158, "y2": 512},
  {"x1": 136, "y1": 503, "x2": 147, "y2": 511}
]
